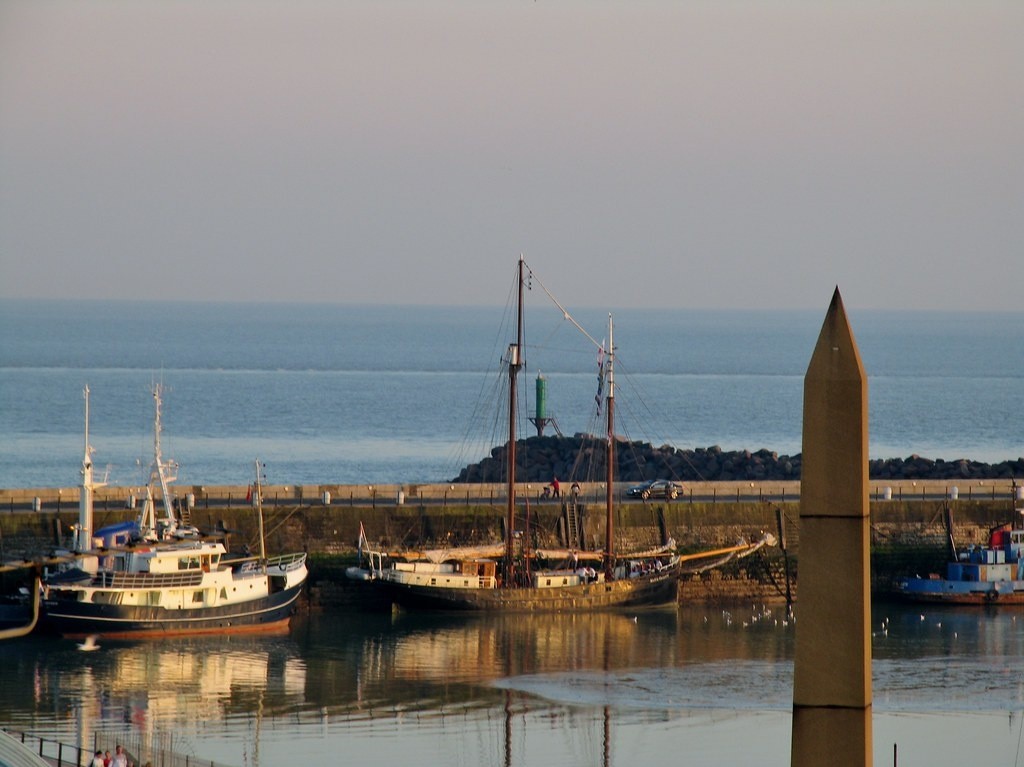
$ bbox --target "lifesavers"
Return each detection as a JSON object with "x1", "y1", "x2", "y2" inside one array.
[{"x1": 984, "y1": 587, "x2": 1000, "y2": 603}]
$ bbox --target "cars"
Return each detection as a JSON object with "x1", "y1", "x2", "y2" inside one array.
[{"x1": 626, "y1": 479, "x2": 683, "y2": 499}]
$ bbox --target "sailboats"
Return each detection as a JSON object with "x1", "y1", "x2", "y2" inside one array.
[{"x1": 347, "y1": 255, "x2": 777, "y2": 613}]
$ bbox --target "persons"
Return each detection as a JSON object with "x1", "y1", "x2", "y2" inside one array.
[
  {"x1": 579, "y1": 564, "x2": 596, "y2": 584},
  {"x1": 570, "y1": 481, "x2": 581, "y2": 501},
  {"x1": 664, "y1": 481, "x2": 672, "y2": 502},
  {"x1": 547, "y1": 477, "x2": 560, "y2": 500},
  {"x1": 572, "y1": 551, "x2": 578, "y2": 573},
  {"x1": 637, "y1": 557, "x2": 663, "y2": 579},
  {"x1": 87, "y1": 743, "x2": 135, "y2": 767}
]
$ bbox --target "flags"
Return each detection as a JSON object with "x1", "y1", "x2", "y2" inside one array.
[{"x1": 591, "y1": 339, "x2": 607, "y2": 415}]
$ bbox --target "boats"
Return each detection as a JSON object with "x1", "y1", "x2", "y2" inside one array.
[
  {"x1": 901, "y1": 477, "x2": 1023, "y2": 605},
  {"x1": 1, "y1": 385, "x2": 316, "y2": 652}
]
